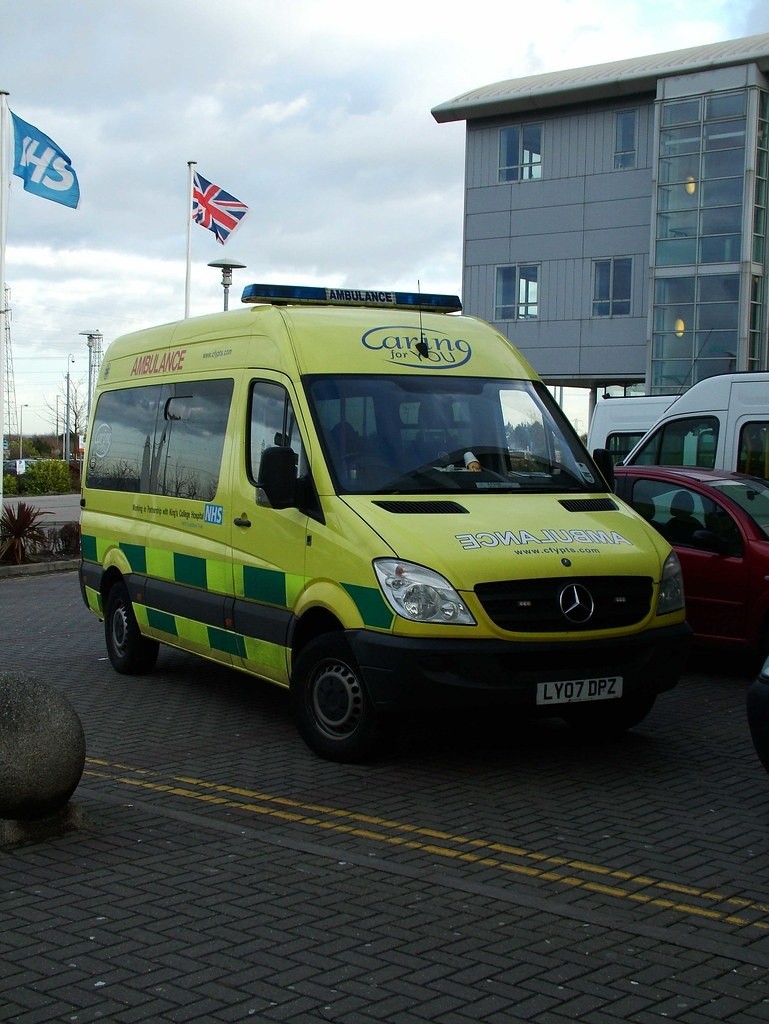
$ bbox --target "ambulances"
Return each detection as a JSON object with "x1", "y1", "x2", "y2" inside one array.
[{"x1": 79, "y1": 284, "x2": 687, "y2": 762}]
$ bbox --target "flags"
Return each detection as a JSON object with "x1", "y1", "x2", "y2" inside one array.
[
  {"x1": 2, "y1": 102, "x2": 81, "y2": 208},
  {"x1": 188, "y1": 170, "x2": 248, "y2": 246}
]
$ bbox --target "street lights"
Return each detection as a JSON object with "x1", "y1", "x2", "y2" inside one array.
[
  {"x1": 20, "y1": 405, "x2": 29, "y2": 459},
  {"x1": 207, "y1": 257, "x2": 247, "y2": 311},
  {"x1": 66, "y1": 353, "x2": 76, "y2": 461}
]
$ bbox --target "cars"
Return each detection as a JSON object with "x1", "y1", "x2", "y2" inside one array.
[{"x1": 613, "y1": 464, "x2": 769, "y2": 658}]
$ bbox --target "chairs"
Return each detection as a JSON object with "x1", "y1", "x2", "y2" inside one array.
[
  {"x1": 661, "y1": 491, "x2": 705, "y2": 546},
  {"x1": 405, "y1": 401, "x2": 458, "y2": 469},
  {"x1": 631, "y1": 495, "x2": 661, "y2": 531}
]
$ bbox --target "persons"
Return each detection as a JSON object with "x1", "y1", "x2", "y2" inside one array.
[
  {"x1": 331, "y1": 425, "x2": 396, "y2": 472},
  {"x1": 412, "y1": 402, "x2": 481, "y2": 473}
]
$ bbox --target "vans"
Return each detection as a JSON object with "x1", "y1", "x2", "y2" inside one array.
[
  {"x1": 586, "y1": 393, "x2": 746, "y2": 484},
  {"x1": 622, "y1": 370, "x2": 769, "y2": 486}
]
